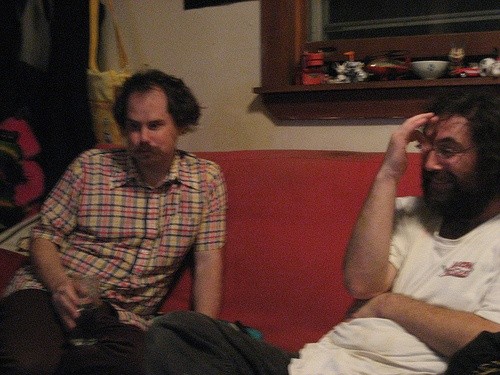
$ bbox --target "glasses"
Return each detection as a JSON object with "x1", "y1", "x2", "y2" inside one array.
[{"x1": 415, "y1": 143, "x2": 476, "y2": 157}]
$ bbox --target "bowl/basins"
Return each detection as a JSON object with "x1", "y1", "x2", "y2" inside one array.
[{"x1": 409, "y1": 61, "x2": 449, "y2": 80}]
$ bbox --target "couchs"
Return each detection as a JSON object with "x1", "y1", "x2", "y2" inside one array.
[{"x1": 1, "y1": 151, "x2": 425, "y2": 354}]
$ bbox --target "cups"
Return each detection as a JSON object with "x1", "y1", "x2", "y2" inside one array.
[{"x1": 68, "y1": 274, "x2": 99, "y2": 346}]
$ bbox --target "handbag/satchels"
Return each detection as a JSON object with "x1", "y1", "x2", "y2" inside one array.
[{"x1": 88, "y1": 62, "x2": 149, "y2": 144}]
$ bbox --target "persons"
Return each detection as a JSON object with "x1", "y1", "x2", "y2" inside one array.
[
  {"x1": 0, "y1": 102, "x2": 45, "y2": 225},
  {"x1": 0, "y1": 71, "x2": 228, "y2": 375},
  {"x1": 140, "y1": 86, "x2": 500, "y2": 375}
]
